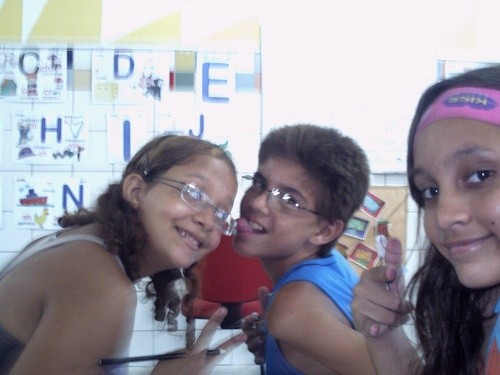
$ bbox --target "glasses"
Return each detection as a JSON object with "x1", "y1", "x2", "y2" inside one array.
[
  {"x1": 241, "y1": 175, "x2": 322, "y2": 216},
  {"x1": 146, "y1": 174, "x2": 237, "y2": 237}
]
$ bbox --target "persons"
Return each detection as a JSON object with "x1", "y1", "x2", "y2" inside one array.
[
  {"x1": 233, "y1": 124, "x2": 378, "y2": 375},
  {"x1": 0, "y1": 133, "x2": 248, "y2": 375},
  {"x1": 351, "y1": 65, "x2": 500, "y2": 375}
]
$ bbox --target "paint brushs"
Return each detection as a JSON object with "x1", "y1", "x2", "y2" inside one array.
[{"x1": 100, "y1": 347, "x2": 227, "y2": 364}]
